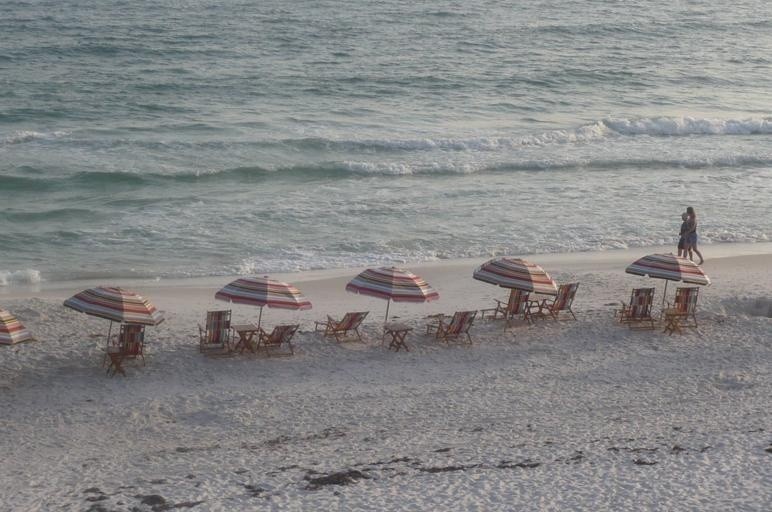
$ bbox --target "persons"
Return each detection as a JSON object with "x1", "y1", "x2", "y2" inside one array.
[
  {"x1": 678, "y1": 212, "x2": 689, "y2": 257},
  {"x1": 679, "y1": 207, "x2": 704, "y2": 265}
]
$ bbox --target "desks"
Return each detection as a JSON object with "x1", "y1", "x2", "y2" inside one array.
[
  {"x1": 661, "y1": 308, "x2": 689, "y2": 336},
  {"x1": 231, "y1": 324, "x2": 259, "y2": 355},
  {"x1": 382, "y1": 322, "x2": 413, "y2": 352}
]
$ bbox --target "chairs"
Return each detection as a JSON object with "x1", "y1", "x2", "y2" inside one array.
[
  {"x1": 254, "y1": 322, "x2": 299, "y2": 358},
  {"x1": 101, "y1": 323, "x2": 145, "y2": 379},
  {"x1": 479, "y1": 282, "x2": 579, "y2": 325},
  {"x1": 196, "y1": 309, "x2": 230, "y2": 355},
  {"x1": 436, "y1": 310, "x2": 477, "y2": 345},
  {"x1": 314, "y1": 310, "x2": 369, "y2": 344},
  {"x1": 659, "y1": 286, "x2": 699, "y2": 329},
  {"x1": 613, "y1": 287, "x2": 656, "y2": 331}
]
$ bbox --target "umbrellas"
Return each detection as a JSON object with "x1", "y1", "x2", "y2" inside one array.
[
  {"x1": 625, "y1": 253, "x2": 711, "y2": 321},
  {"x1": 345, "y1": 267, "x2": 440, "y2": 344},
  {"x1": 473, "y1": 258, "x2": 558, "y2": 324},
  {"x1": 0, "y1": 307, "x2": 38, "y2": 346},
  {"x1": 64, "y1": 285, "x2": 165, "y2": 369},
  {"x1": 214, "y1": 275, "x2": 312, "y2": 345}
]
